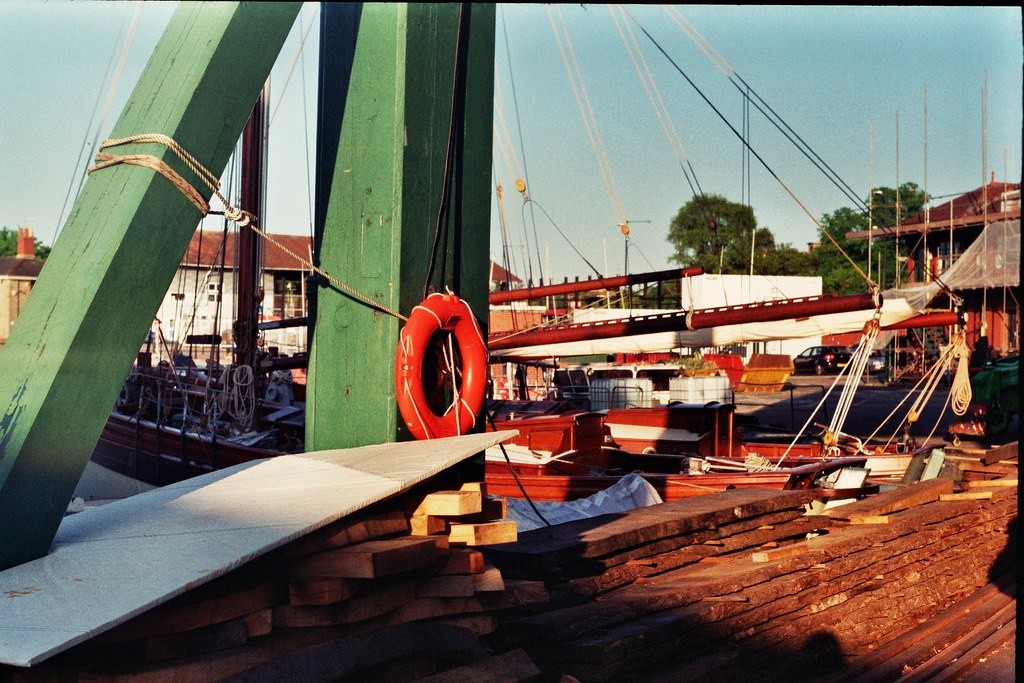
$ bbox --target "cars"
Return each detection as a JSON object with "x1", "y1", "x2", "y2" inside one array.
[
  {"x1": 791, "y1": 345, "x2": 856, "y2": 376},
  {"x1": 848, "y1": 343, "x2": 887, "y2": 374}
]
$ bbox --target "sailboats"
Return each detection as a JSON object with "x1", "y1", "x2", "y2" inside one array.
[{"x1": 52, "y1": 0, "x2": 1024, "y2": 501}]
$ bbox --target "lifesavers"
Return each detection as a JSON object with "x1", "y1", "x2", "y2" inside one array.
[
  {"x1": 393, "y1": 291, "x2": 491, "y2": 441},
  {"x1": 499, "y1": 376, "x2": 519, "y2": 400}
]
